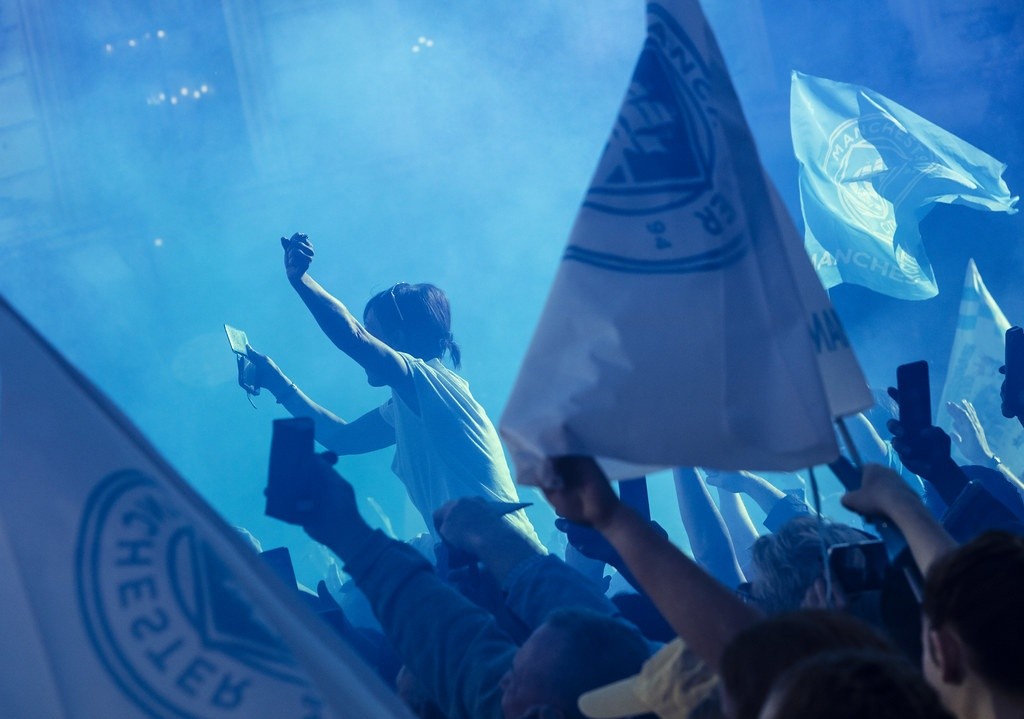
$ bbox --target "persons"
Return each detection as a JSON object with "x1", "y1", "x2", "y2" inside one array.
[
  {"x1": 225, "y1": 232, "x2": 550, "y2": 564},
  {"x1": 263, "y1": 326, "x2": 1024, "y2": 718}
]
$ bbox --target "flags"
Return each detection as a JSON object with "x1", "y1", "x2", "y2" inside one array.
[
  {"x1": 790, "y1": 68, "x2": 1022, "y2": 301},
  {"x1": 934, "y1": 257, "x2": 1024, "y2": 482},
  {"x1": 0, "y1": 299, "x2": 412, "y2": 719},
  {"x1": 498, "y1": 1, "x2": 878, "y2": 486}
]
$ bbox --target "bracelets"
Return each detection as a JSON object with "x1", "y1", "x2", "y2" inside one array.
[
  {"x1": 275, "y1": 382, "x2": 297, "y2": 405},
  {"x1": 988, "y1": 455, "x2": 1002, "y2": 471}
]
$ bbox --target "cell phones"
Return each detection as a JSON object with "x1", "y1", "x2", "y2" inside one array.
[
  {"x1": 897, "y1": 360, "x2": 933, "y2": 459},
  {"x1": 828, "y1": 540, "x2": 893, "y2": 592},
  {"x1": 940, "y1": 481, "x2": 1024, "y2": 546},
  {"x1": 224, "y1": 325, "x2": 251, "y2": 356},
  {"x1": 1003, "y1": 326, "x2": 1024, "y2": 416},
  {"x1": 265, "y1": 418, "x2": 314, "y2": 524}
]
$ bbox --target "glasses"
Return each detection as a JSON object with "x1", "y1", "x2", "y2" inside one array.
[{"x1": 382, "y1": 282, "x2": 410, "y2": 320}]
